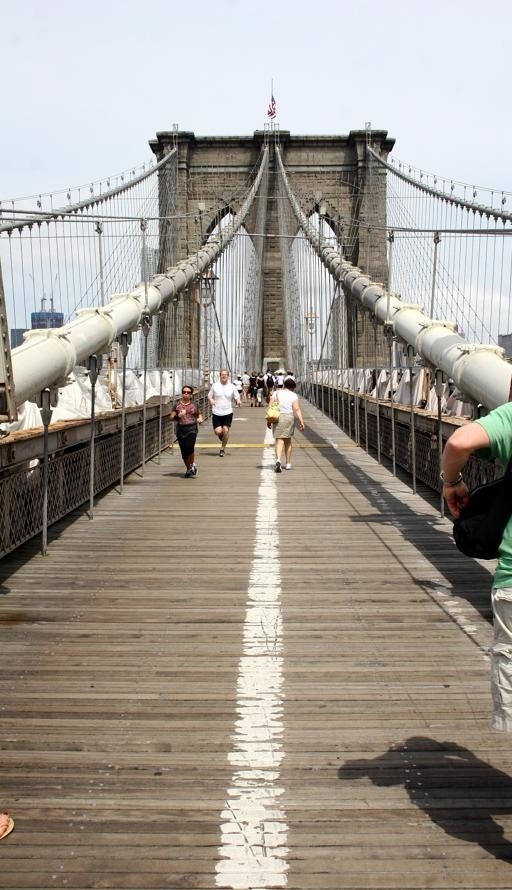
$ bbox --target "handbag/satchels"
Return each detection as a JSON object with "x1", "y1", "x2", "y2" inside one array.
[
  {"x1": 265, "y1": 402, "x2": 280, "y2": 423},
  {"x1": 452, "y1": 472, "x2": 511, "y2": 560}
]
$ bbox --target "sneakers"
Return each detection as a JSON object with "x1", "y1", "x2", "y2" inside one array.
[
  {"x1": 246, "y1": 398, "x2": 265, "y2": 407},
  {"x1": 285, "y1": 463, "x2": 293, "y2": 470},
  {"x1": 187, "y1": 467, "x2": 198, "y2": 478},
  {"x1": 275, "y1": 459, "x2": 282, "y2": 472},
  {"x1": 186, "y1": 469, "x2": 191, "y2": 478},
  {"x1": 220, "y1": 449, "x2": 226, "y2": 457}
]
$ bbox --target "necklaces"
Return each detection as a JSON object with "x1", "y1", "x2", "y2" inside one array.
[{"x1": 179, "y1": 399, "x2": 191, "y2": 406}]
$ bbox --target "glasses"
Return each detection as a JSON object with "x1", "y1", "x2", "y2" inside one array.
[{"x1": 182, "y1": 390, "x2": 192, "y2": 394}]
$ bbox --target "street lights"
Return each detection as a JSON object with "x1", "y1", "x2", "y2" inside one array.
[
  {"x1": 293, "y1": 334, "x2": 307, "y2": 377},
  {"x1": 191, "y1": 268, "x2": 220, "y2": 389},
  {"x1": 239, "y1": 335, "x2": 254, "y2": 376},
  {"x1": 305, "y1": 305, "x2": 320, "y2": 373}
]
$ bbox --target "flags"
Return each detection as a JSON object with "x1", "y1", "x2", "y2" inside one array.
[{"x1": 264, "y1": 78, "x2": 278, "y2": 121}]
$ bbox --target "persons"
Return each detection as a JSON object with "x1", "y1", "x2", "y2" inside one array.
[
  {"x1": 207, "y1": 369, "x2": 241, "y2": 457},
  {"x1": 266, "y1": 378, "x2": 306, "y2": 473},
  {"x1": 232, "y1": 369, "x2": 295, "y2": 408},
  {"x1": 437, "y1": 382, "x2": 510, "y2": 740},
  {"x1": 168, "y1": 385, "x2": 205, "y2": 478},
  {"x1": 1, "y1": 813, "x2": 16, "y2": 840}
]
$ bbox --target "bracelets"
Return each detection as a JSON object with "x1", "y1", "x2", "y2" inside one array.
[{"x1": 439, "y1": 470, "x2": 465, "y2": 489}]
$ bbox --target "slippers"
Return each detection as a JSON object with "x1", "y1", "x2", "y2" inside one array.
[{"x1": 1, "y1": 813, "x2": 14, "y2": 840}]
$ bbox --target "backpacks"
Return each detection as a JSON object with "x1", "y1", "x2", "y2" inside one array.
[{"x1": 267, "y1": 373, "x2": 274, "y2": 388}]
